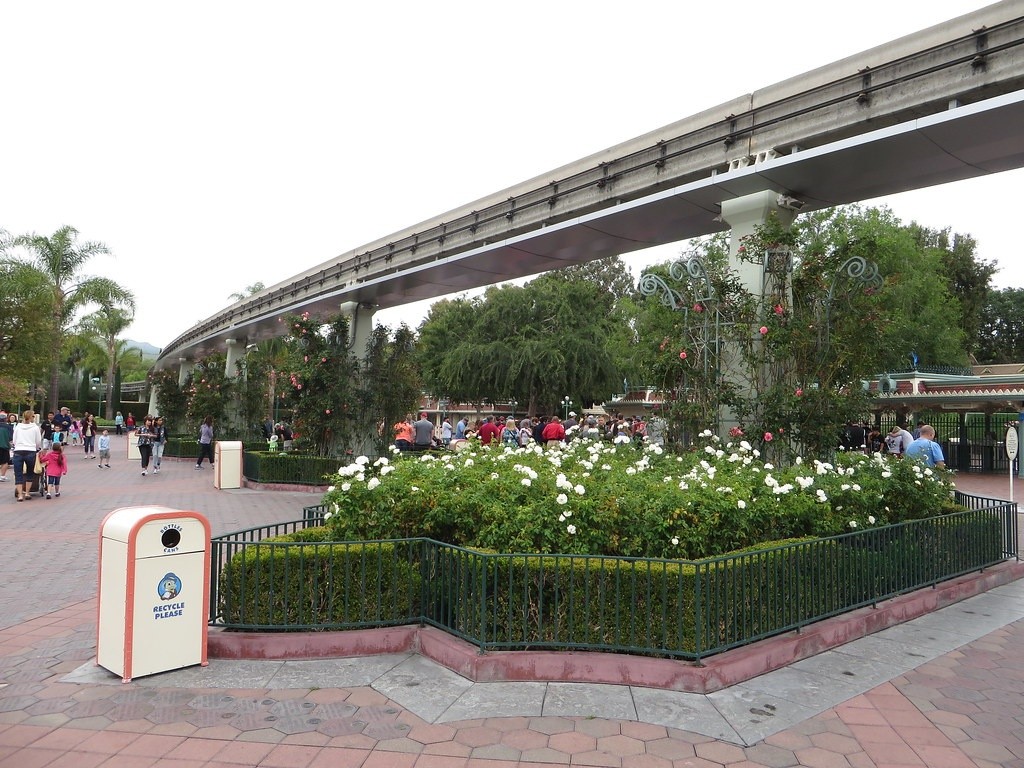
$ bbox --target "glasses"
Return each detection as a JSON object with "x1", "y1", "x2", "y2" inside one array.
[{"x1": 598, "y1": 419, "x2": 604, "y2": 422}]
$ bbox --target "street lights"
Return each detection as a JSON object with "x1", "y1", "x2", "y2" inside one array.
[{"x1": 561, "y1": 396, "x2": 573, "y2": 422}]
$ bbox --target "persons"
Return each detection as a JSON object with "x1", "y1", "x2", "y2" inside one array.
[
  {"x1": 840, "y1": 421, "x2": 944, "y2": 482},
  {"x1": 378, "y1": 411, "x2": 670, "y2": 453},
  {"x1": 135, "y1": 415, "x2": 167, "y2": 475},
  {"x1": 195, "y1": 415, "x2": 215, "y2": 470},
  {"x1": 115, "y1": 412, "x2": 136, "y2": 435},
  {"x1": 0, "y1": 407, "x2": 111, "y2": 501},
  {"x1": 262, "y1": 412, "x2": 295, "y2": 441}
]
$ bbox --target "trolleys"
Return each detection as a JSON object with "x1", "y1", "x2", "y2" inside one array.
[{"x1": 11, "y1": 447, "x2": 48, "y2": 498}]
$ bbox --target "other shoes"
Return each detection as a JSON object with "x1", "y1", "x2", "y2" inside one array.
[
  {"x1": 83, "y1": 445, "x2": 85, "y2": 448},
  {"x1": 61, "y1": 442, "x2": 69, "y2": 446},
  {"x1": 120, "y1": 432, "x2": 123, "y2": 435},
  {"x1": 55, "y1": 493, "x2": 60, "y2": 497},
  {"x1": 8, "y1": 465, "x2": 11, "y2": 469},
  {"x1": 46, "y1": 495, "x2": 52, "y2": 500},
  {"x1": 88, "y1": 444, "x2": 90, "y2": 447},
  {"x1": 116, "y1": 432, "x2": 118, "y2": 435},
  {"x1": 24, "y1": 494, "x2": 32, "y2": 500},
  {"x1": 72, "y1": 443, "x2": 78, "y2": 447},
  {"x1": 17, "y1": 497, "x2": 24, "y2": 502},
  {"x1": 79, "y1": 443, "x2": 83, "y2": 446}
]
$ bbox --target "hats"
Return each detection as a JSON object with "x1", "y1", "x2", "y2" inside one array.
[
  {"x1": 270, "y1": 435, "x2": 278, "y2": 442},
  {"x1": 421, "y1": 412, "x2": 427, "y2": 417},
  {"x1": 506, "y1": 415, "x2": 514, "y2": 419},
  {"x1": 0, "y1": 413, "x2": 8, "y2": 419},
  {"x1": 568, "y1": 411, "x2": 577, "y2": 417},
  {"x1": 498, "y1": 416, "x2": 504, "y2": 420},
  {"x1": 275, "y1": 423, "x2": 280, "y2": 427},
  {"x1": 589, "y1": 420, "x2": 598, "y2": 425},
  {"x1": 61, "y1": 407, "x2": 70, "y2": 411},
  {"x1": 551, "y1": 416, "x2": 561, "y2": 423}
]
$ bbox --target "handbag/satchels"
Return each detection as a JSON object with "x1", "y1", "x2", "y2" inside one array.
[
  {"x1": 196, "y1": 438, "x2": 201, "y2": 445},
  {"x1": 34, "y1": 450, "x2": 43, "y2": 475},
  {"x1": 91, "y1": 429, "x2": 97, "y2": 436}
]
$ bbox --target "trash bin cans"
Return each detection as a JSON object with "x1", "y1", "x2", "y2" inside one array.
[
  {"x1": 212, "y1": 439, "x2": 243, "y2": 491},
  {"x1": 127, "y1": 429, "x2": 143, "y2": 462},
  {"x1": 448, "y1": 438, "x2": 469, "y2": 452},
  {"x1": 943, "y1": 437, "x2": 970, "y2": 468},
  {"x1": 92, "y1": 504, "x2": 213, "y2": 683}
]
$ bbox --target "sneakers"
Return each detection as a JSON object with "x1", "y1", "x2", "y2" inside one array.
[
  {"x1": 0, "y1": 475, "x2": 10, "y2": 482},
  {"x1": 90, "y1": 455, "x2": 96, "y2": 459},
  {"x1": 97, "y1": 464, "x2": 103, "y2": 469},
  {"x1": 153, "y1": 467, "x2": 160, "y2": 474},
  {"x1": 194, "y1": 464, "x2": 204, "y2": 470},
  {"x1": 212, "y1": 464, "x2": 215, "y2": 469},
  {"x1": 141, "y1": 470, "x2": 149, "y2": 476},
  {"x1": 84, "y1": 455, "x2": 88, "y2": 459},
  {"x1": 104, "y1": 463, "x2": 110, "y2": 468}
]
